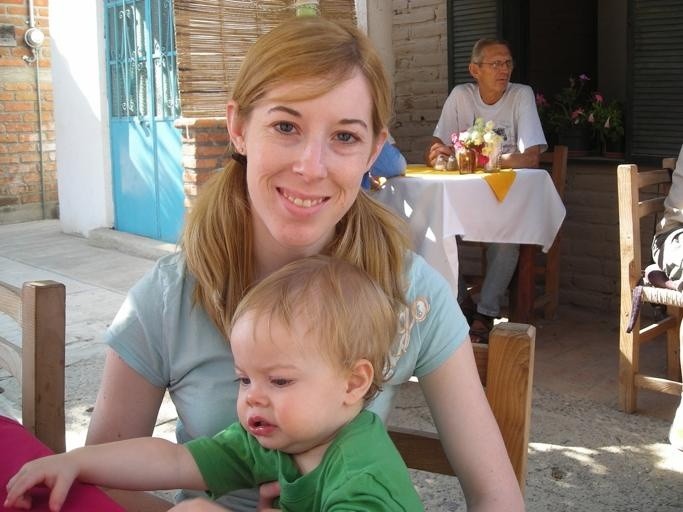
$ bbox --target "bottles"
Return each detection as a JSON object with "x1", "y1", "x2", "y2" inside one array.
[
  {"x1": 433, "y1": 153, "x2": 456, "y2": 171},
  {"x1": 457, "y1": 144, "x2": 476, "y2": 175}
]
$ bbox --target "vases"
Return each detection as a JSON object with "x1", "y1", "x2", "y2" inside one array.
[
  {"x1": 595, "y1": 125, "x2": 630, "y2": 159},
  {"x1": 551, "y1": 123, "x2": 593, "y2": 163},
  {"x1": 452, "y1": 144, "x2": 478, "y2": 176}
]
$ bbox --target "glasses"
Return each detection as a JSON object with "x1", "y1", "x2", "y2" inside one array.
[{"x1": 471, "y1": 58, "x2": 519, "y2": 72}]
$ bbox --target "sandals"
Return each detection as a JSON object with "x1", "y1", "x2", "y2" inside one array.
[{"x1": 470, "y1": 313, "x2": 497, "y2": 345}]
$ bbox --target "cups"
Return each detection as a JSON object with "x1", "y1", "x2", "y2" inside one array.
[{"x1": 483, "y1": 142, "x2": 502, "y2": 173}]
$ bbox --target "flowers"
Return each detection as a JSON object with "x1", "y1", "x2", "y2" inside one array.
[
  {"x1": 436, "y1": 108, "x2": 504, "y2": 161},
  {"x1": 529, "y1": 64, "x2": 606, "y2": 141},
  {"x1": 570, "y1": 88, "x2": 630, "y2": 139}
]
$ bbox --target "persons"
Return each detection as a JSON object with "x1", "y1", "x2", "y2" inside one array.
[
  {"x1": 80, "y1": 14, "x2": 526, "y2": 512},
  {"x1": 423, "y1": 34, "x2": 549, "y2": 340},
  {"x1": 649, "y1": 141, "x2": 683, "y2": 452},
  {"x1": 0, "y1": 253, "x2": 426, "y2": 512}
]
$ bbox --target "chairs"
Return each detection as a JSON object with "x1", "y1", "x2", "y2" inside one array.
[
  {"x1": 611, "y1": 155, "x2": 683, "y2": 417},
  {"x1": 0, "y1": 276, "x2": 66, "y2": 468},
  {"x1": 350, "y1": 317, "x2": 538, "y2": 507},
  {"x1": 445, "y1": 140, "x2": 569, "y2": 326}
]
